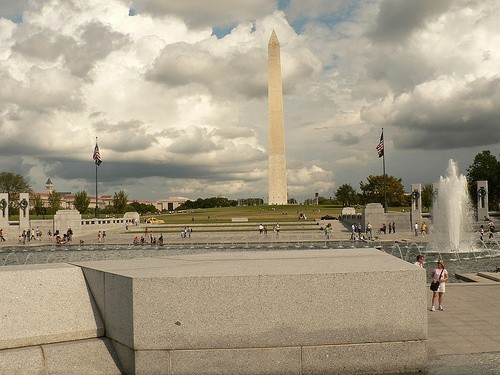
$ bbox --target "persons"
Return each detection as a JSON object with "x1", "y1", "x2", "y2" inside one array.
[
  {"x1": 133, "y1": 236, "x2": 139, "y2": 246},
  {"x1": 350, "y1": 221, "x2": 396, "y2": 242},
  {"x1": 150, "y1": 230, "x2": 153, "y2": 243},
  {"x1": 98, "y1": 231, "x2": 106, "y2": 243},
  {"x1": 259, "y1": 222, "x2": 280, "y2": 236},
  {"x1": 487, "y1": 220, "x2": 495, "y2": 240},
  {"x1": 479, "y1": 225, "x2": 484, "y2": 244},
  {"x1": 55, "y1": 228, "x2": 73, "y2": 245},
  {"x1": 323, "y1": 223, "x2": 333, "y2": 239},
  {"x1": 46, "y1": 229, "x2": 52, "y2": 242},
  {"x1": 0, "y1": 229, "x2": 6, "y2": 241},
  {"x1": 414, "y1": 221, "x2": 426, "y2": 237},
  {"x1": 18, "y1": 226, "x2": 42, "y2": 245},
  {"x1": 414, "y1": 255, "x2": 425, "y2": 268},
  {"x1": 430, "y1": 259, "x2": 449, "y2": 311},
  {"x1": 140, "y1": 236, "x2": 145, "y2": 246},
  {"x1": 125, "y1": 220, "x2": 129, "y2": 230},
  {"x1": 144, "y1": 227, "x2": 149, "y2": 237},
  {"x1": 180, "y1": 225, "x2": 193, "y2": 240}
]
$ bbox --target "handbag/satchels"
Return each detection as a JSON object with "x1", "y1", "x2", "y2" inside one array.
[{"x1": 430, "y1": 280, "x2": 440, "y2": 291}]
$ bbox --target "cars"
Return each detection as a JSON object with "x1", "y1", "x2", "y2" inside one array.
[{"x1": 145, "y1": 217, "x2": 165, "y2": 224}]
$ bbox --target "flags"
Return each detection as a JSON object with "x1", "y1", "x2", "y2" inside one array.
[
  {"x1": 376, "y1": 132, "x2": 384, "y2": 157},
  {"x1": 93, "y1": 144, "x2": 103, "y2": 166}
]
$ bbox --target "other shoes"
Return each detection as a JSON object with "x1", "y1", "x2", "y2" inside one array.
[
  {"x1": 439, "y1": 305, "x2": 443, "y2": 310},
  {"x1": 431, "y1": 306, "x2": 435, "y2": 311}
]
